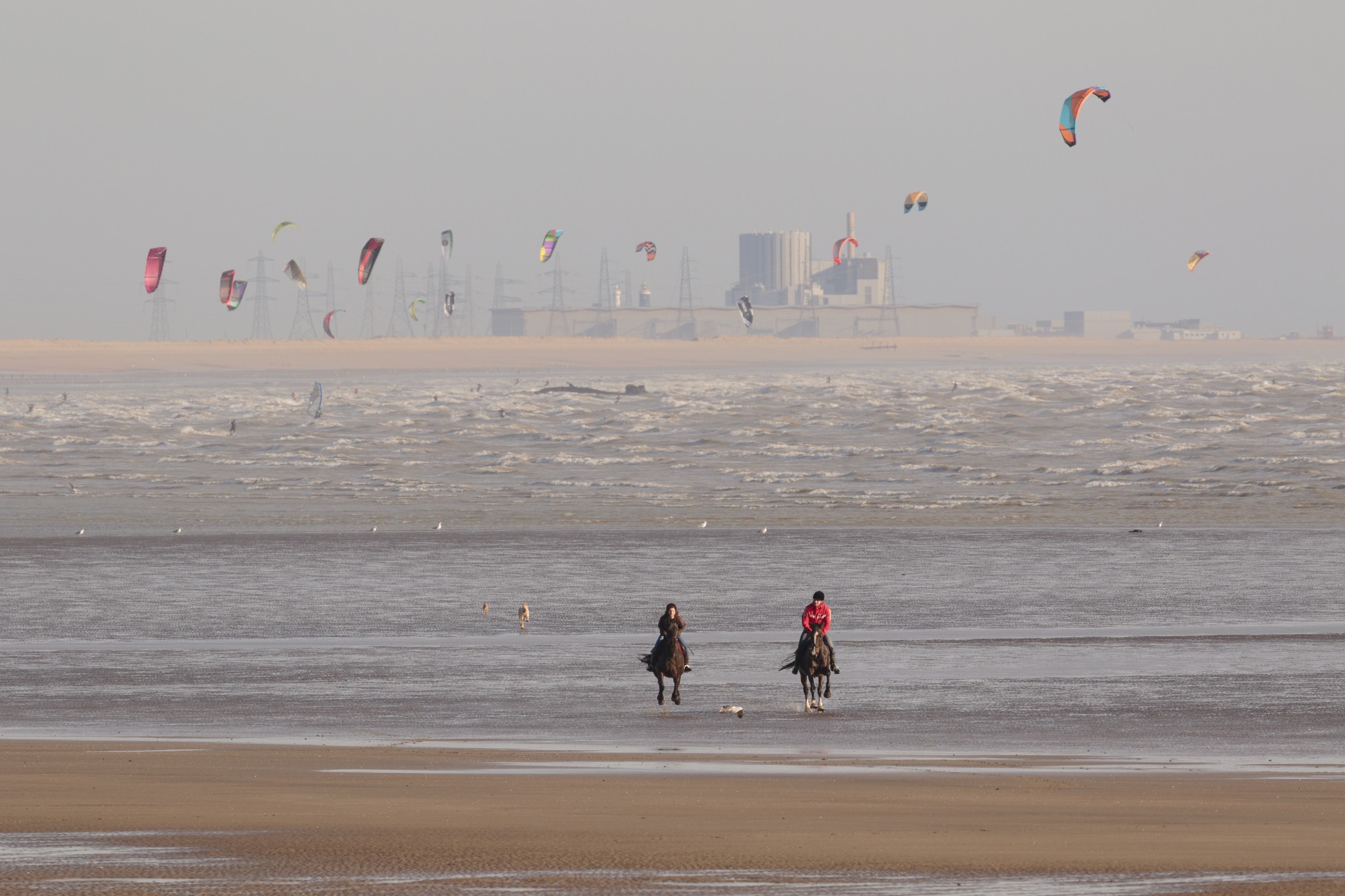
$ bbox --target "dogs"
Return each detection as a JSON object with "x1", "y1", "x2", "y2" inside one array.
[
  {"x1": 518, "y1": 603, "x2": 530, "y2": 629},
  {"x1": 482, "y1": 603, "x2": 489, "y2": 613}
]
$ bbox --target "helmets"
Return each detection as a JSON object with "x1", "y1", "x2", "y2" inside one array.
[
  {"x1": 812, "y1": 591, "x2": 824, "y2": 601},
  {"x1": 666, "y1": 603, "x2": 677, "y2": 611}
]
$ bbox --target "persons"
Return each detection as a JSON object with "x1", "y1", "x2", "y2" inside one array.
[
  {"x1": 792, "y1": 591, "x2": 840, "y2": 675},
  {"x1": 6, "y1": 357, "x2": 1276, "y2": 438},
  {"x1": 647, "y1": 603, "x2": 691, "y2": 672}
]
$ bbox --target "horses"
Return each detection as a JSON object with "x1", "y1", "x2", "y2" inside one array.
[
  {"x1": 779, "y1": 621, "x2": 840, "y2": 715},
  {"x1": 637, "y1": 620, "x2": 689, "y2": 707}
]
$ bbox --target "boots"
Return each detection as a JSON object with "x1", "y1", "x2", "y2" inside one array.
[
  {"x1": 684, "y1": 653, "x2": 692, "y2": 673},
  {"x1": 830, "y1": 653, "x2": 840, "y2": 674},
  {"x1": 792, "y1": 651, "x2": 800, "y2": 675},
  {"x1": 647, "y1": 652, "x2": 656, "y2": 672}
]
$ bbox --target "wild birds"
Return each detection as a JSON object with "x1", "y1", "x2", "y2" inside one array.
[
  {"x1": 432, "y1": 522, "x2": 443, "y2": 531},
  {"x1": 195, "y1": 518, "x2": 205, "y2": 523},
  {"x1": 718, "y1": 704, "x2": 745, "y2": 719},
  {"x1": 696, "y1": 519, "x2": 709, "y2": 529},
  {"x1": 75, "y1": 528, "x2": 86, "y2": 536},
  {"x1": 69, "y1": 483, "x2": 81, "y2": 493},
  {"x1": 173, "y1": 526, "x2": 182, "y2": 535},
  {"x1": 254, "y1": 478, "x2": 264, "y2": 485},
  {"x1": 370, "y1": 526, "x2": 378, "y2": 534},
  {"x1": 757, "y1": 526, "x2": 768, "y2": 534}
]
